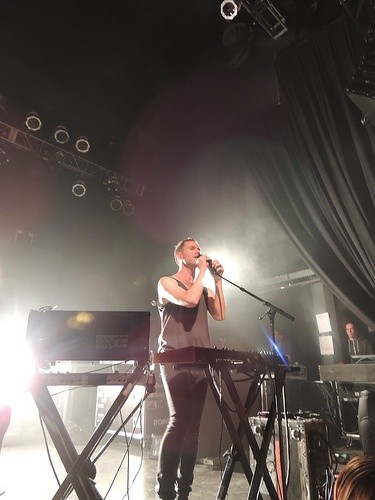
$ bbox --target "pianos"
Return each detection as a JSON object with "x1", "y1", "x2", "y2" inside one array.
[{"x1": 152, "y1": 345, "x2": 288, "y2": 364}]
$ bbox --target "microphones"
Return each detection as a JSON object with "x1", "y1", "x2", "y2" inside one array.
[{"x1": 197, "y1": 254, "x2": 217, "y2": 272}]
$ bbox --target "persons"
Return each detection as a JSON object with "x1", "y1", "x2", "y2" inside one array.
[
  {"x1": 155, "y1": 236, "x2": 226, "y2": 500},
  {"x1": 269, "y1": 330, "x2": 299, "y2": 411},
  {"x1": 345, "y1": 322, "x2": 375, "y2": 449},
  {"x1": 333, "y1": 455, "x2": 375, "y2": 500}
]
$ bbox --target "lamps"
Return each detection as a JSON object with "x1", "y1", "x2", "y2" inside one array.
[
  {"x1": 75, "y1": 136, "x2": 91, "y2": 153},
  {"x1": 25, "y1": 111, "x2": 42, "y2": 131},
  {"x1": 71, "y1": 176, "x2": 87, "y2": 197},
  {"x1": 53, "y1": 125, "x2": 70, "y2": 144},
  {"x1": 220, "y1": 0, "x2": 242, "y2": 20}
]
